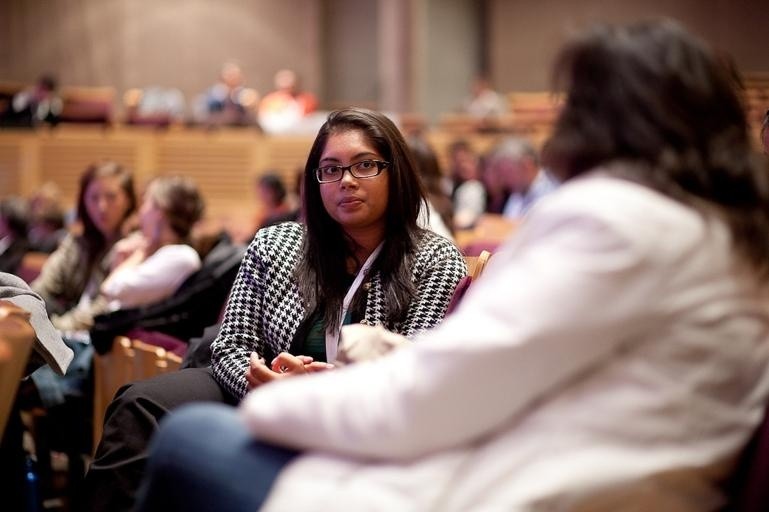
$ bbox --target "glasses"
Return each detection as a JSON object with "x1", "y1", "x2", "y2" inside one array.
[{"x1": 316, "y1": 159, "x2": 391, "y2": 183}]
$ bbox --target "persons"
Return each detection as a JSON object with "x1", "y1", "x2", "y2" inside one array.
[
  {"x1": 2, "y1": 57, "x2": 316, "y2": 129},
  {"x1": 409, "y1": 136, "x2": 563, "y2": 219},
  {"x1": 76, "y1": 107, "x2": 465, "y2": 511},
  {"x1": 131, "y1": 13, "x2": 769, "y2": 512},
  {"x1": 1, "y1": 166, "x2": 309, "y2": 512}
]
